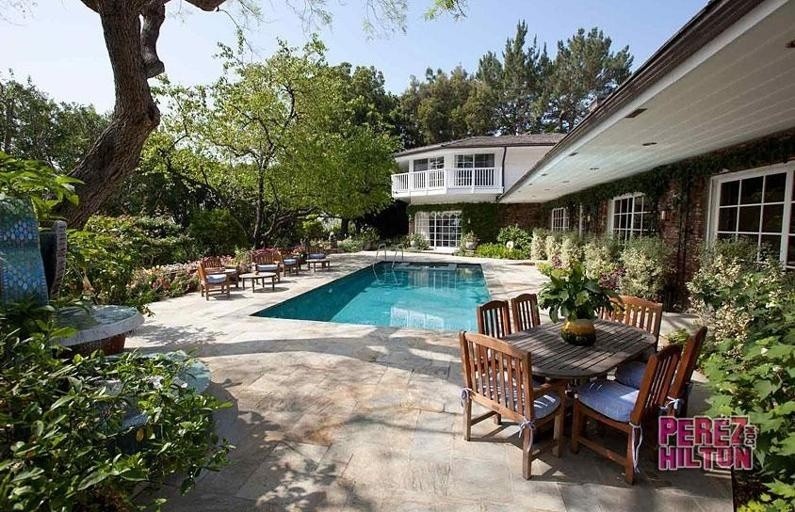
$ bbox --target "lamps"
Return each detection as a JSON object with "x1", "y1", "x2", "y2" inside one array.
[
  {"x1": 661, "y1": 209, "x2": 673, "y2": 221},
  {"x1": 587, "y1": 213, "x2": 592, "y2": 222}
]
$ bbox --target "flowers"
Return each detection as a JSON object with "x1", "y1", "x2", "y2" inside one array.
[{"x1": 538, "y1": 253, "x2": 625, "y2": 323}]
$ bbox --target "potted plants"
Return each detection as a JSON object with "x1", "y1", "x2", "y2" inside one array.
[
  {"x1": 497, "y1": 223, "x2": 532, "y2": 249},
  {"x1": 359, "y1": 223, "x2": 380, "y2": 250},
  {"x1": 455, "y1": 230, "x2": 478, "y2": 256},
  {"x1": 703, "y1": 335, "x2": 795, "y2": 512},
  {"x1": 400, "y1": 233, "x2": 428, "y2": 250}
]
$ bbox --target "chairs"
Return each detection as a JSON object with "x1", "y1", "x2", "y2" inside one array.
[
  {"x1": 459, "y1": 293, "x2": 708, "y2": 485},
  {"x1": 195, "y1": 245, "x2": 331, "y2": 301}
]
